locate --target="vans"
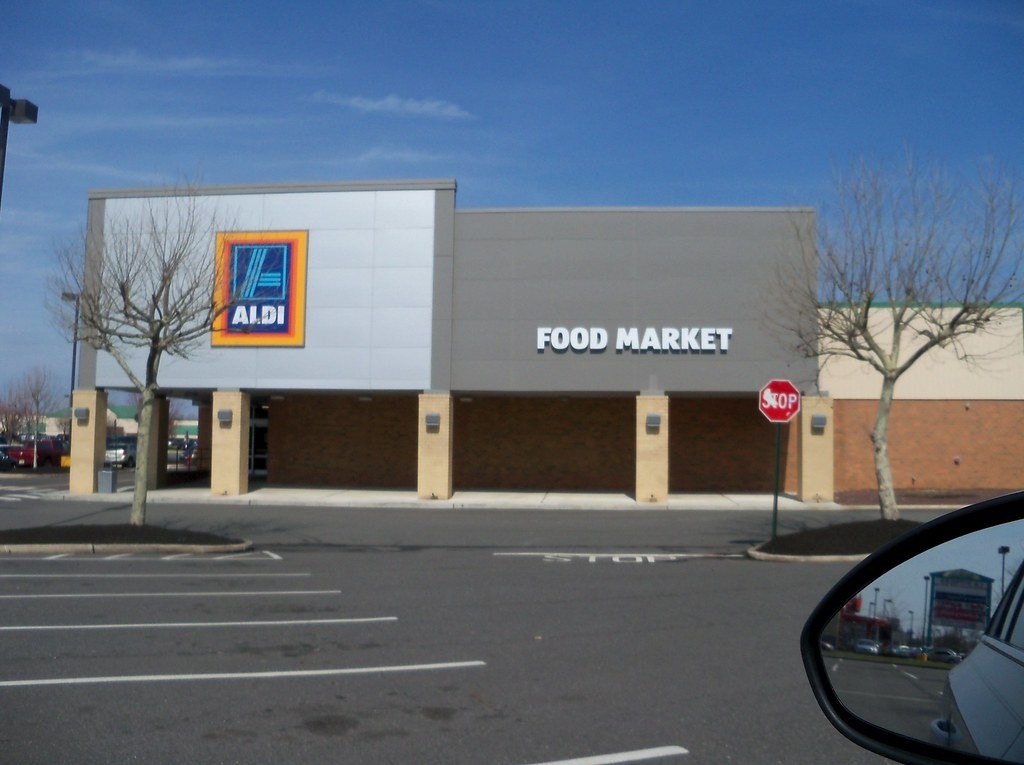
[168,438,198,462]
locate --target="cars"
[106,435,138,468]
[854,639,879,654]
[0,428,70,475]
[889,643,964,662]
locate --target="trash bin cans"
[97,469,117,493]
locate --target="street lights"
[999,546,1010,599]
[61,292,79,408]
[922,576,930,644]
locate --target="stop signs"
[758,380,800,422]
